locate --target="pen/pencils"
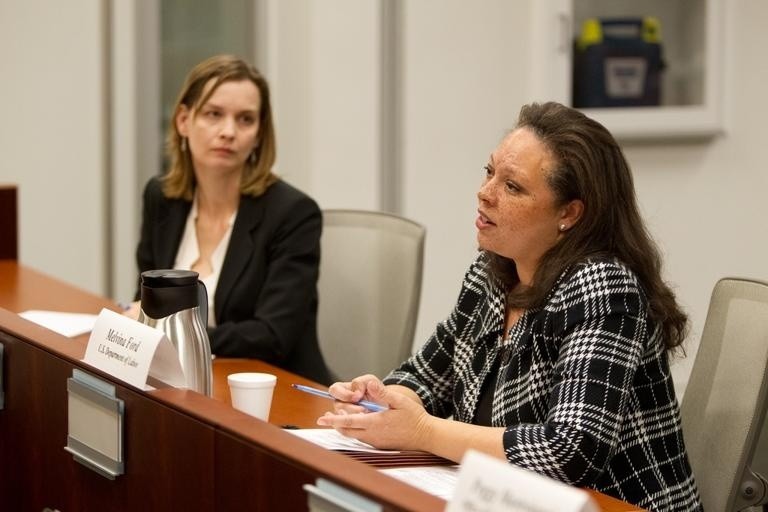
[289,383,387,412]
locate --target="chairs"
[318,209,427,387]
[680,275,768,511]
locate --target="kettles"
[136,269,215,398]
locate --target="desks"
[0,258,647,512]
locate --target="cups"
[227,373,278,423]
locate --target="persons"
[122,53,333,387]
[317,102,703,512]
